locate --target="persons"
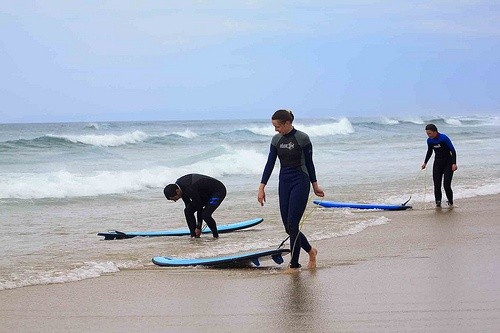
[258,110,325,274]
[164,174,227,240]
[420,123,457,210]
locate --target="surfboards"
[97,217,264,240]
[313,200,412,210]
[152,249,290,266]
